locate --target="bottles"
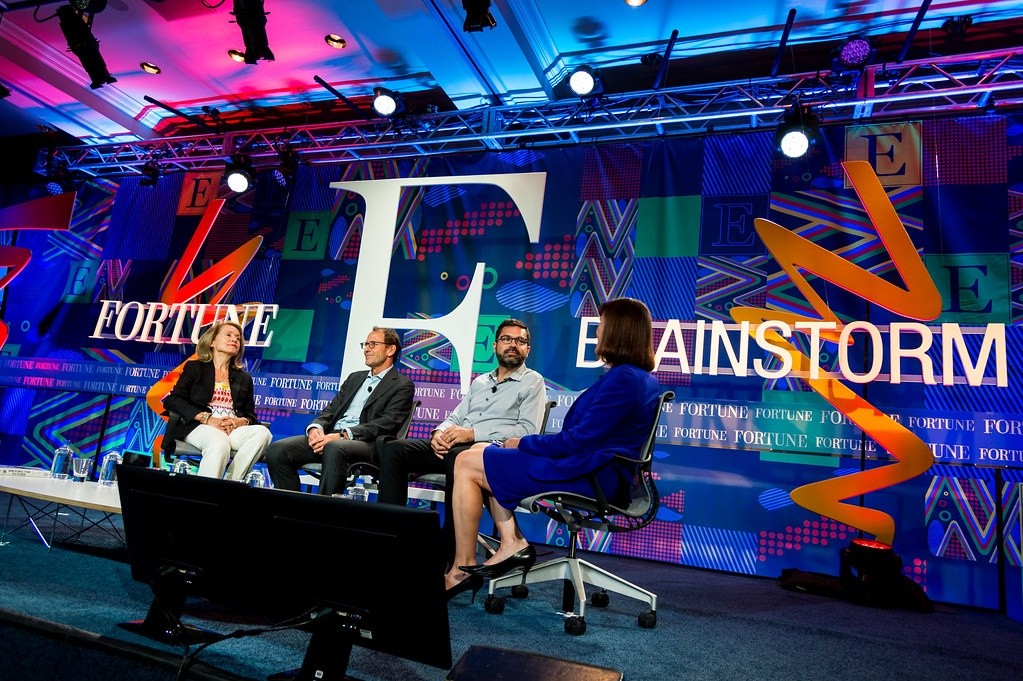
[174,456,192,475]
[348,478,369,502]
[98,447,122,490]
[50,439,74,479]
[245,464,265,488]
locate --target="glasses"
[360,341,390,350]
[496,335,528,346]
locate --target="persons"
[161,322,273,480]
[267,327,416,496]
[378,319,546,532]
[446,298,660,606]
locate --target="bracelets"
[200,411,211,424]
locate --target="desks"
[0,465,127,553]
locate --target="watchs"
[339,433,345,439]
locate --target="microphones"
[230,375,236,383]
[368,386,372,393]
[492,386,497,392]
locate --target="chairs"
[484,390,675,634]
[414,400,558,572]
[301,401,422,501]
[164,395,271,476]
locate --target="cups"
[332,493,353,500]
[72,458,91,482]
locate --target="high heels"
[447,574,484,604]
[459,544,537,585]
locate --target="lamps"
[227,163,253,192]
[272,157,298,188]
[373,86,398,116]
[774,111,809,159]
[44,174,70,195]
[569,64,599,95]
[139,160,161,188]
[839,36,871,65]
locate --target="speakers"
[447,644,625,681]
